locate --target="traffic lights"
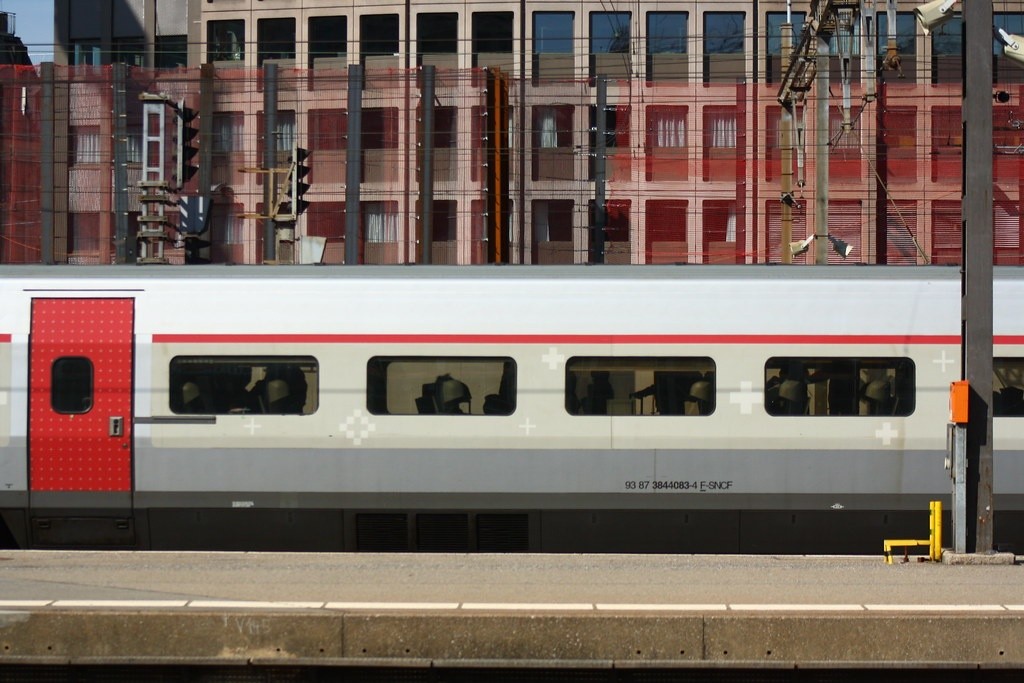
[171,98,200,190]
[287,140,311,220]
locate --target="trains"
[0,263,1024,555]
